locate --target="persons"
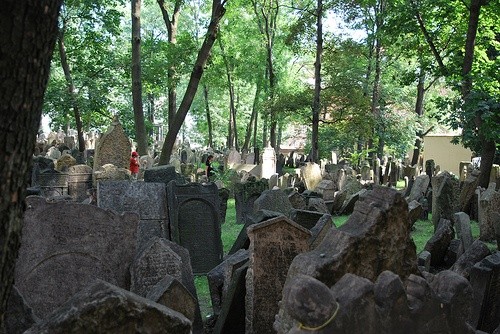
[205,155,214,184]
[130,151,141,180]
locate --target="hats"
[132,152,138,156]
